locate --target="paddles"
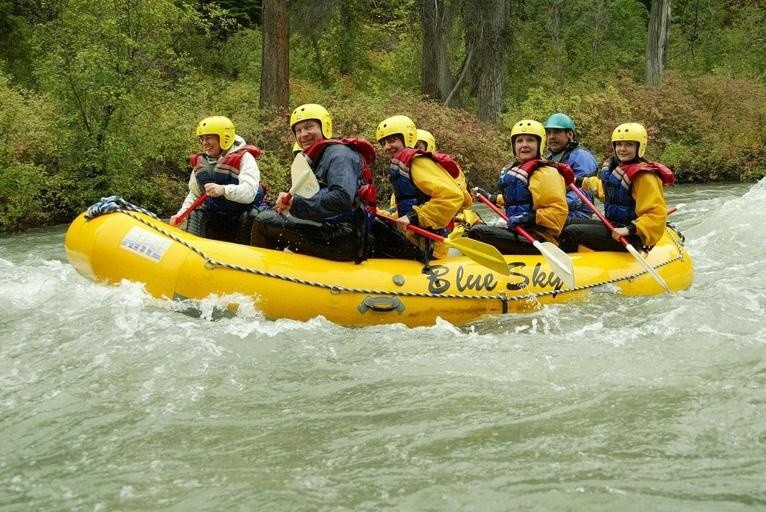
[476,194,574,289]
[376,213,509,274]
[569,182,669,294]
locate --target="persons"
[252,104,378,262]
[169,115,265,244]
[376,114,466,262]
[542,112,597,226]
[461,120,568,256]
[556,123,674,254]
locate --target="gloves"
[507,211,529,230]
[470,186,496,206]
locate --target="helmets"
[376,115,418,149]
[510,119,547,158]
[544,112,575,133]
[417,129,435,155]
[290,103,332,158]
[611,122,648,159]
[196,116,235,151]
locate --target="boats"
[63,200,697,329]
